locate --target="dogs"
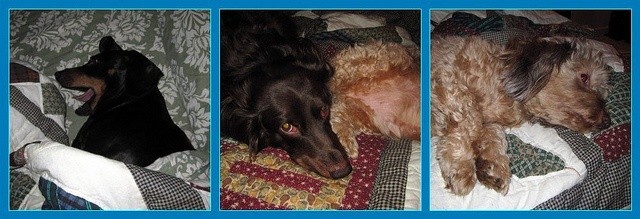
[54,35,196,167]
[220,10,353,180]
[324,31,615,200]
